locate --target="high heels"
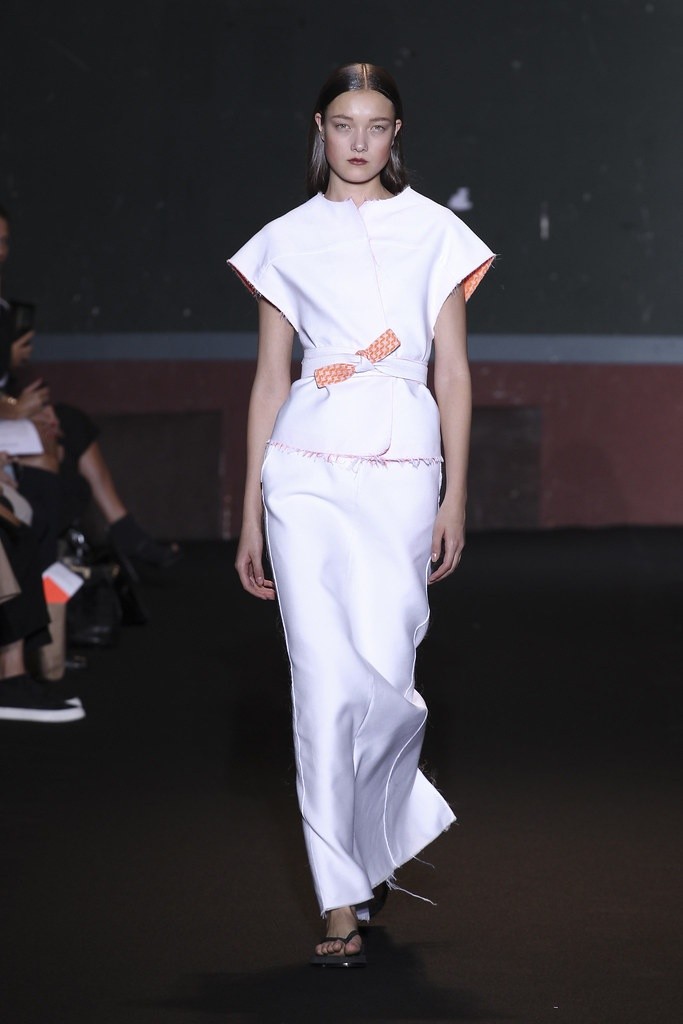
[101,513,182,584]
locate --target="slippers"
[309,929,367,968]
[366,881,389,917]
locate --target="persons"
[228,61,495,971]
[0,217,180,723]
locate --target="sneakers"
[0,672,86,723]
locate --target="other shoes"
[119,606,151,624]
